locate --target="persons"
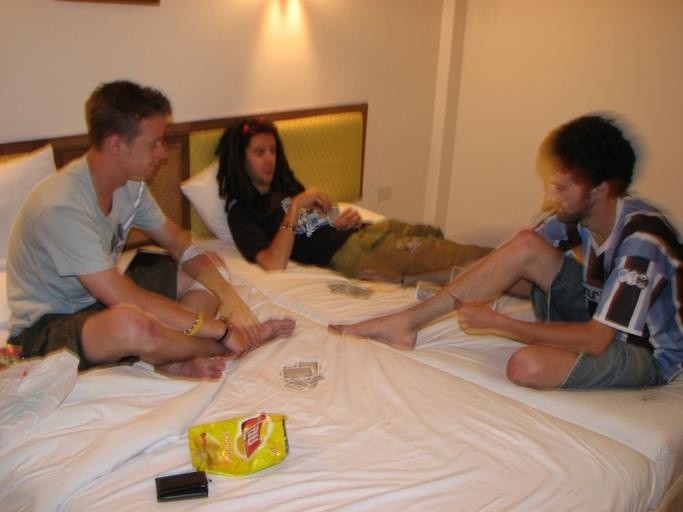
[214,117,540,298]
[5,79,296,382]
[326,112,682,391]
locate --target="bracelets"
[214,322,231,342]
[278,222,297,234]
[182,311,206,337]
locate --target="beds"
[0,101,682,511]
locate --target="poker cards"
[448,290,463,302]
[450,267,463,281]
[327,281,376,300]
[327,204,339,226]
[279,360,325,391]
[415,280,442,300]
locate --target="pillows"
[182,157,233,242]
[0,143,57,272]
